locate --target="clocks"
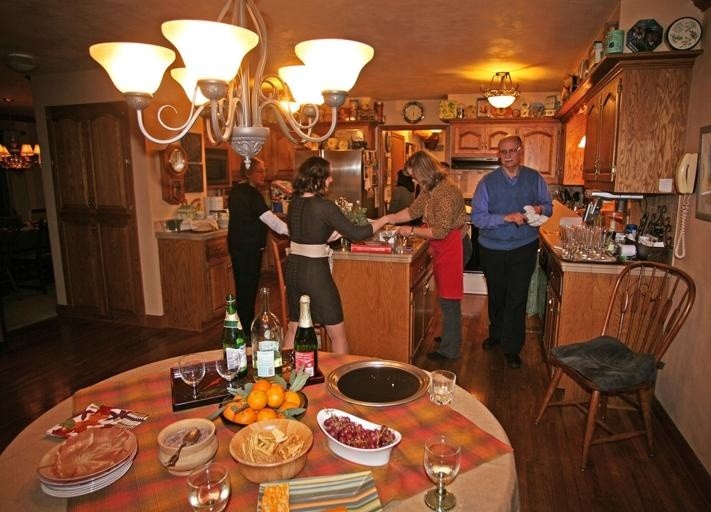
[157,142,191,205]
[401,101,426,125]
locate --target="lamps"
[85,0,377,172]
[478,71,524,111]
[0,116,42,171]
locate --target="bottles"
[163,199,227,232]
[407,232,417,251]
[570,21,625,89]
[605,198,641,261]
[294,295,318,377]
[553,188,582,211]
[251,288,284,381]
[310,99,385,151]
[220,294,249,377]
[437,96,558,117]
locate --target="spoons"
[166,427,203,469]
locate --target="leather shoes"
[504,351,522,369]
[482,335,501,351]
[427,352,449,360]
[434,336,443,344]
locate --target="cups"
[187,463,233,511]
[431,371,456,404]
[559,222,606,260]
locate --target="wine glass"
[215,349,242,393]
[381,224,394,244]
[423,435,463,510]
[180,353,208,399]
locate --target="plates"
[628,19,664,52]
[256,470,382,512]
[38,403,148,497]
[667,18,703,52]
[219,385,310,434]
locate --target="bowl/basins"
[229,418,314,482]
[156,416,218,475]
[318,405,403,467]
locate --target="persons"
[469,134,554,369]
[283,156,394,354]
[385,147,467,361]
[394,157,418,194]
[224,154,290,335]
[438,160,452,175]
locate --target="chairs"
[527,259,698,474]
[271,239,325,349]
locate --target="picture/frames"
[694,125,711,224]
[405,141,414,159]
[385,154,394,186]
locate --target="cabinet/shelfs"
[558,87,587,191]
[202,110,298,189]
[157,214,290,335]
[320,237,440,363]
[534,244,667,395]
[582,49,702,201]
[516,112,565,187]
[446,118,515,162]
[298,116,383,150]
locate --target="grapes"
[324,417,395,449]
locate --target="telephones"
[674,153,698,194]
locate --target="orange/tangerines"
[223,380,300,424]
